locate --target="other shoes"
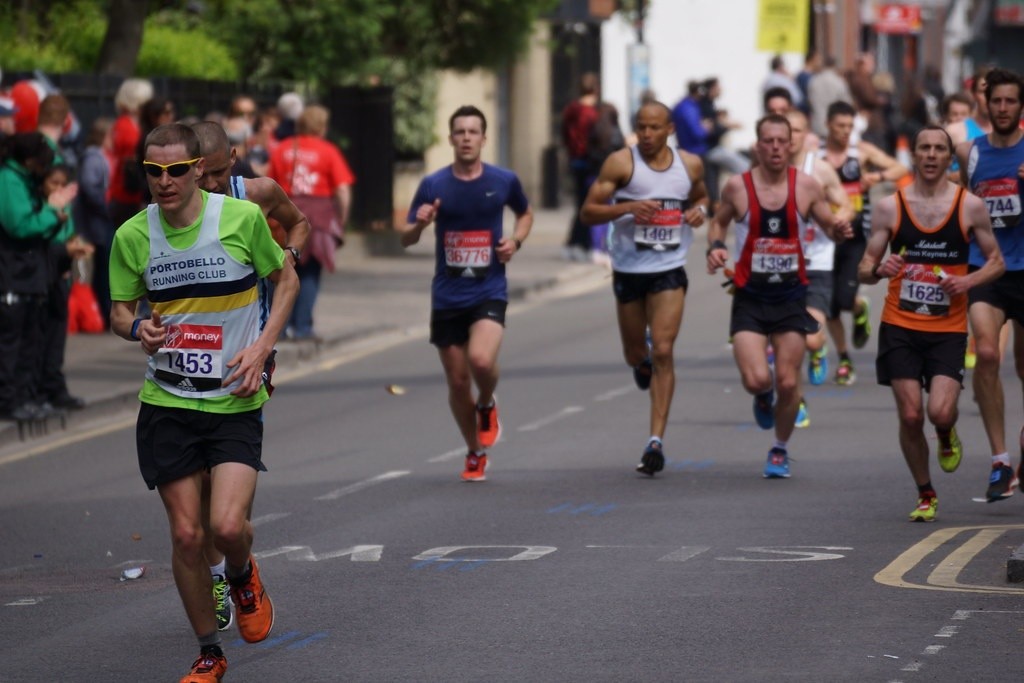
[6,396,68,425]
[49,385,89,409]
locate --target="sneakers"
[461,448,489,482]
[1016,463,1024,493]
[210,573,233,632]
[853,296,872,349]
[762,448,798,478]
[752,363,776,430]
[908,496,938,523]
[793,397,810,427]
[224,552,275,643]
[937,423,963,473]
[630,329,653,390]
[808,346,828,386]
[831,359,856,385]
[179,654,228,683]
[475,392,501,449]
[634,445,665,478]
[985,461,1020,500]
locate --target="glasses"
[142,157,201,177]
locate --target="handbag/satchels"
[66,256,107,333]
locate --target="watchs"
[696,204,708,217]
[509,238,521,250]
[872,263,883,279]
[711,241,725,248]
[284,246,302,268]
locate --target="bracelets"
[131,318,144,341]
[880,172,884,181]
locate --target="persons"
[856,126,1006,525]
[108,118,314,683]
[952,68,1024,504]
[579,99,710,476]
[0,77,357,423]
[560,71,751,264]
[706,116,855,480]
[749,50,1009,428]
[397,104,534,483]
